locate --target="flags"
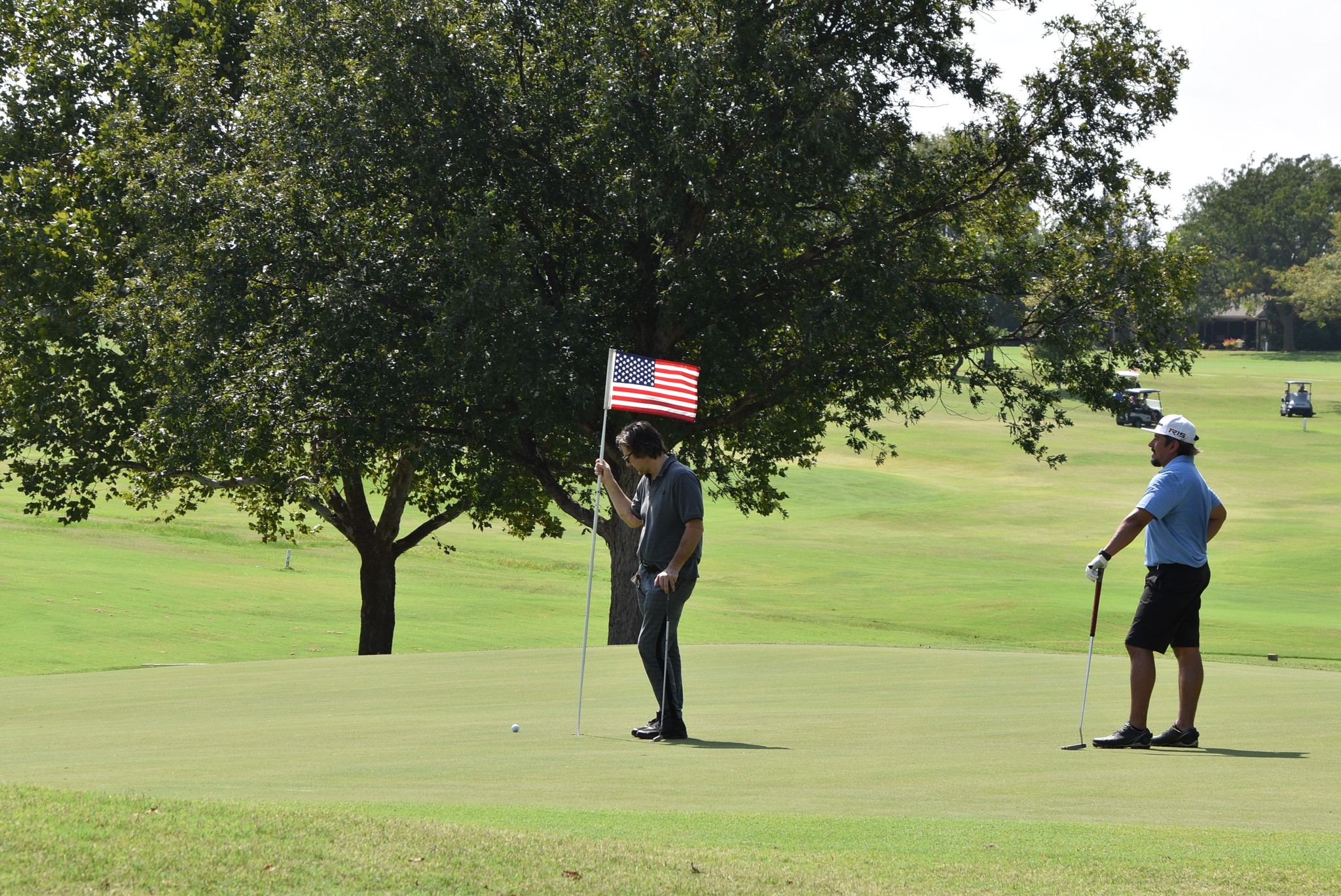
[610,350,701,423]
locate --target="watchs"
[1098,548,1112,561]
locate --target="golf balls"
[511,724,520,732]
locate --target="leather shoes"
[631,719,688,739]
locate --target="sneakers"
[1152,723,1200,747]
[1092,722,1153,750]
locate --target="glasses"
[622,452,633,463]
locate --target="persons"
[1298,385,1308,393]
[1139,392,1158,425]
[1085,413,1227,750]
[595,422,705,740]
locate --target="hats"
[1141,414,1197,444]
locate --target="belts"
[640,561,661,572]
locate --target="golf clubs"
[652,582,672,743]
[1061,567,1105,750]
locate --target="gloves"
[1085,555,1117,574]
